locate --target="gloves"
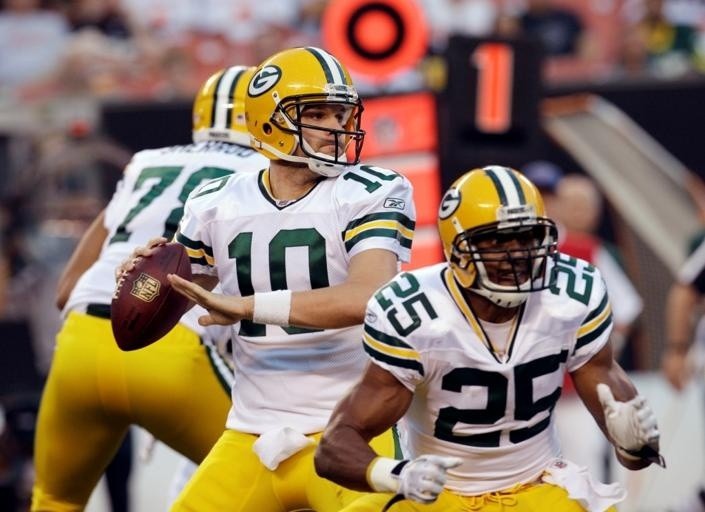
[367,454,464,503]
[596,383,661,461]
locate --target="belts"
[87,304,111,319]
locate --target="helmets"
[192,64,258,148]
[245,47,365,177]
[438,165,560,308]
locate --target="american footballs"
[111,243,192,350]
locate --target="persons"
[1,0,705,511]
